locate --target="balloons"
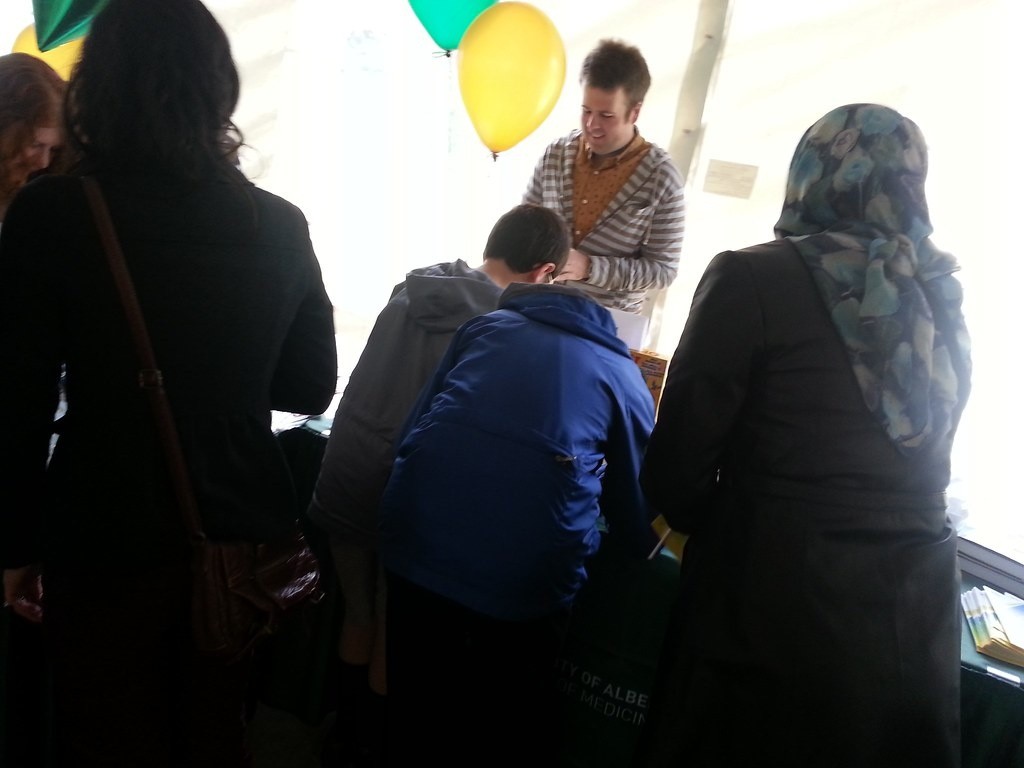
[11,19,85,82]
[408,0,500,58]
[458,1,567,162]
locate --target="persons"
[301,204,570,768]
[380,283,655,768]
[522,40,686,355]
[0,0,338,768]
[638,104,973,768]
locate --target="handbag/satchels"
[198,533,320,655]
